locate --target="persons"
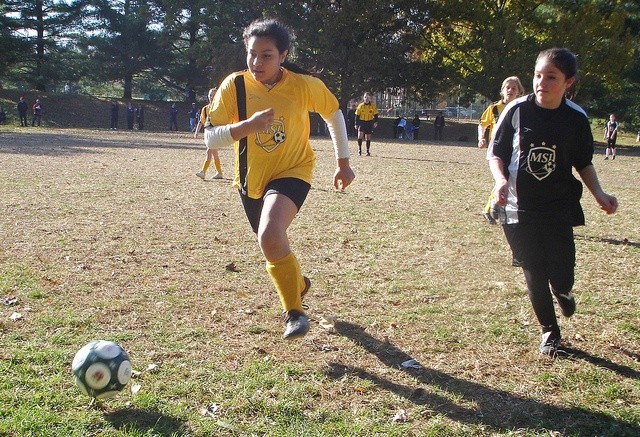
[188,103,196,132]
[204,18,356,340]
[17,96,28,127]
[65,82,70,95]
[195,109,200,122]
[0,108,6,124]
[168,102,178,131]
[193,88,224,180]
[477,75,525,225]
[434,112,445,140]
[486,47,618,356]
[109,99,118,130]
[387,108,393,116]
[136,103,144,130]
[603,114,618,160]
[354,91,379,156]
[412,114,420,140]
[398,117,407,140]
[126,101,134,130]
[392,117,400,139]
[31,99,42,127]
[347,104,357,137]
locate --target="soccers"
[70,340,131,400]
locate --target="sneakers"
[282,309,309,341]
[211,173,224,179]
[552,287,575,317]
[540,332,561,355]
[196,171,205,179]
[300,276,311,309]
